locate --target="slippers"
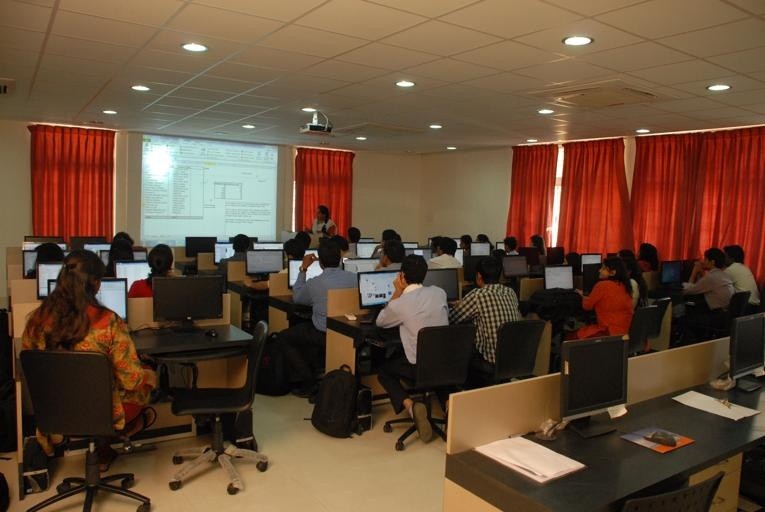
[312,364,357,438]
[253,332,292,396]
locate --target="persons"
[566,243,656,337]
[220,234,250,264]
[30,232,134,279]
[267,228,405,287]
[684,248,735,344]
[426,234,544,268]
[375,254,449,441]
[21,247,159,471]
[128,244,173,299]
[448,258,519,389]
[723,244,761,312]
[313,205,336,238]
[270,240,358,399]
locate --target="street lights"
[531,289,583,310]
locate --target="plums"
[298,122,333,138]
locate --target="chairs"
[411,402,431,442]
[97,446,117,469]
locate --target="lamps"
[473,318,545,384]
[170,319,268,495]
[701,289,750,335]
[17,348,150,512]
[385,325,480,451]
[651,297,672,326]
[618,469,725,512]
[623,303,658,356]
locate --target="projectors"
[298,122,333,138]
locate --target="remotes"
[643,428,677,449]
[206,328,219,340]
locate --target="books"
[472,436,587,484]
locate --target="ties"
[22,241,221,331]
[562,333,630,439]
[730,311,765,383]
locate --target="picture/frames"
[230,280,265,306]
[445,381,763,511]
[267,295,312,334]
[126,323,255,359]
[326,315,389,408]
[174,258,194,277]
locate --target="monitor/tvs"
[661,259,683,285]
[403,238,463,267]
[245,249,284,282]
[343,259,381,275]
[547,246,565,265]
[358,270,402,325]
[288,259,323,289]
[681,259,695,283]
[496,242,506,251]
[185,231,382,258]
[730,311,765,383]
[501,255,528,278]
[472,436,587,484]
[562,333,630,439]
[579,254,602,273]
[518,246,541,271]
[22,241,221,331]
[544,266,574,290]
[470,242,491,256]
[582,264,603,297]
